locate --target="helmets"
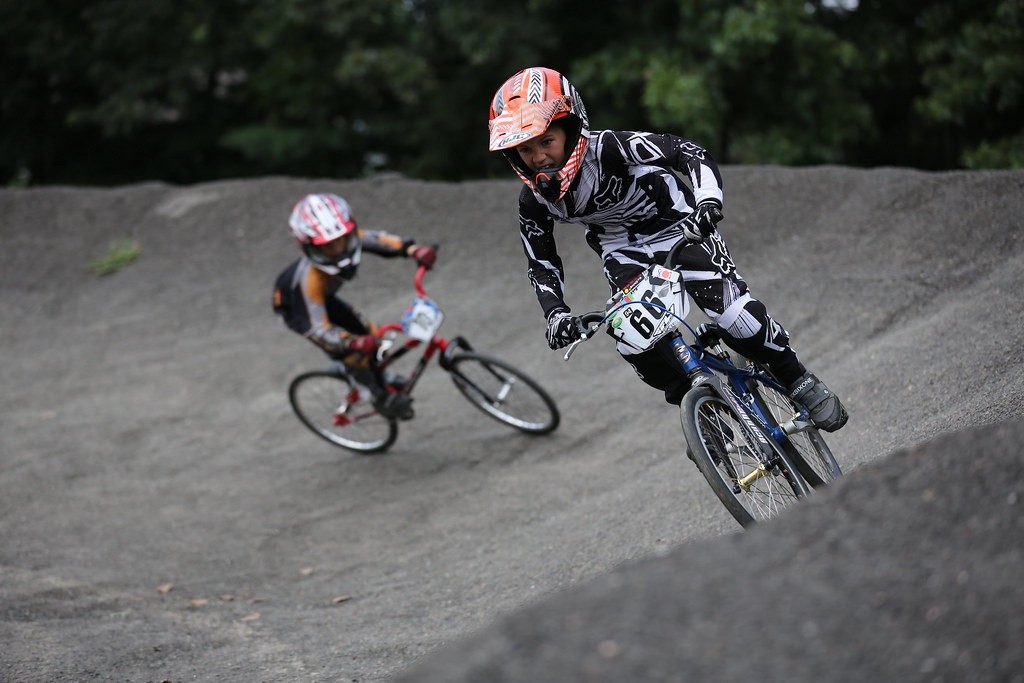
[289,193,361,280]
[489,67,590,205]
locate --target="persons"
[268,191,437,421]
[486,64,849,471]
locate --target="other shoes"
[384,375,407,391]
[372,391,415,421]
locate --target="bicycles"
[287,241,562,456]
[555,205,845,532]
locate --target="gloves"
[680,203,719,246]
[545,307,581,352]
[350,335,379,356]
[408,245,437,271]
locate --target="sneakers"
[686,412,734,460]
[788,370,841,433]
